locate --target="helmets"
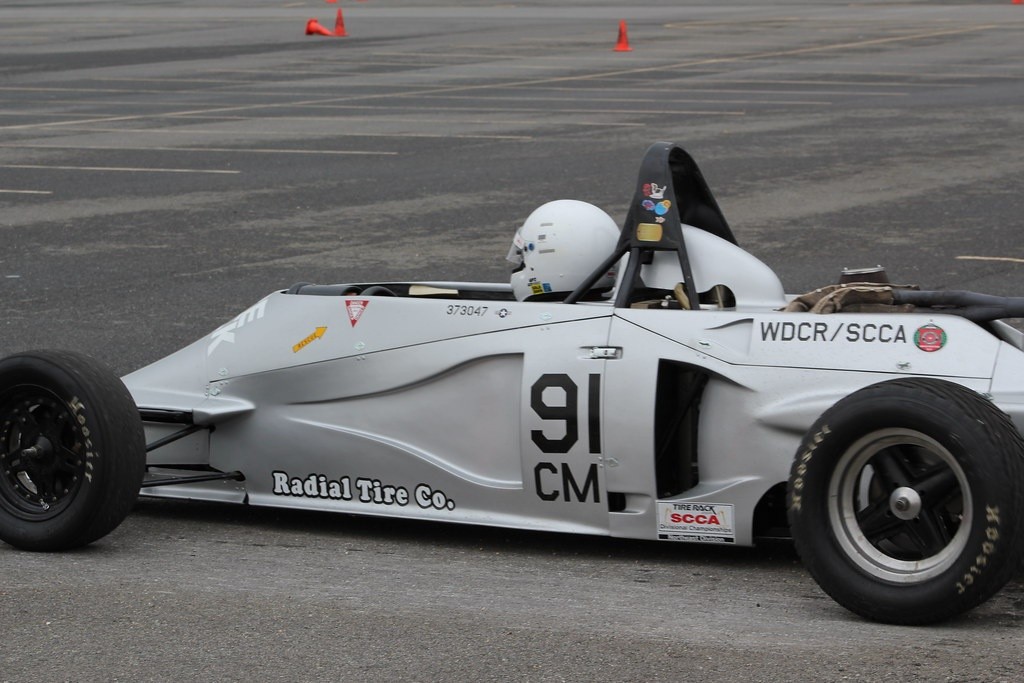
[511,199,621,302]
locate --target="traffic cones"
[613,18,633,53]
[304,19,332,37]
[330,6,350,38]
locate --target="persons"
[505,200,622,302]
